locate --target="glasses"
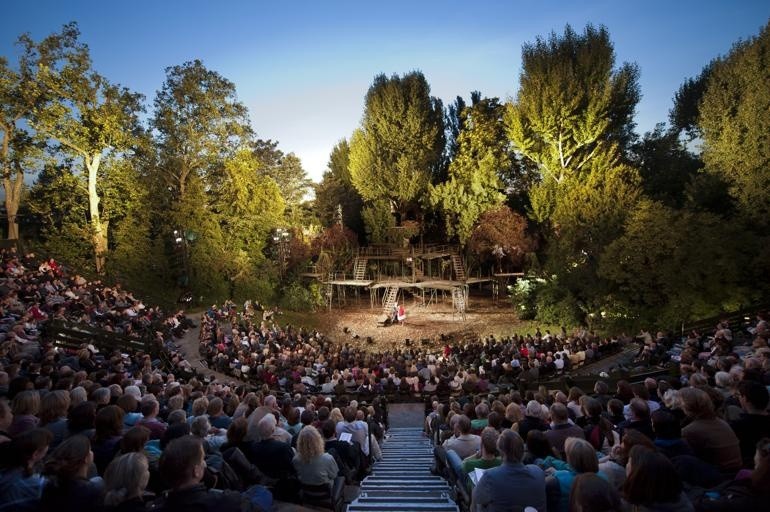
[735,393,742,398]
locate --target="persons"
[421,383,770,511]
[390,300,399,324]
[631,308,770,384]
[307,325,631,384]
[0,237,199,511]
[199,373,390,512]
[396,302,407,327]
[198,300,307,373]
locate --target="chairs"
[298,476,345,512]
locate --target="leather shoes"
[429,465,449,480]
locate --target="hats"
[565,437,598,463]
[240,487,273,512]
